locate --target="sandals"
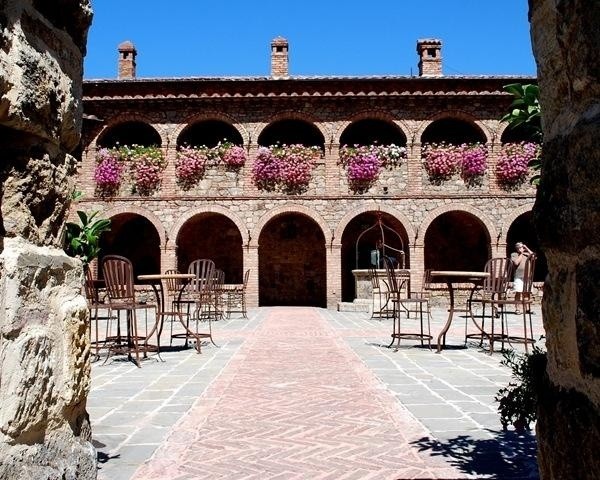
[515,310,521,315]
[526,309,535,314]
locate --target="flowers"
[423,142,540,189]
[92,142,166,195]
[335,139,409,194]
[249,141,328,193]
[174,141,245,189]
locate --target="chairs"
[79,259,115,359]
[100,256,166,368]
[461,255,513,356]
[371,248,434,351]
[162,268,255,320]
[498,254,539,353]
[175,258,219,354]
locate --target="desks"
[426,267,490,354]
[141,273,198,355]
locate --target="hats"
[515,241,523,248]
